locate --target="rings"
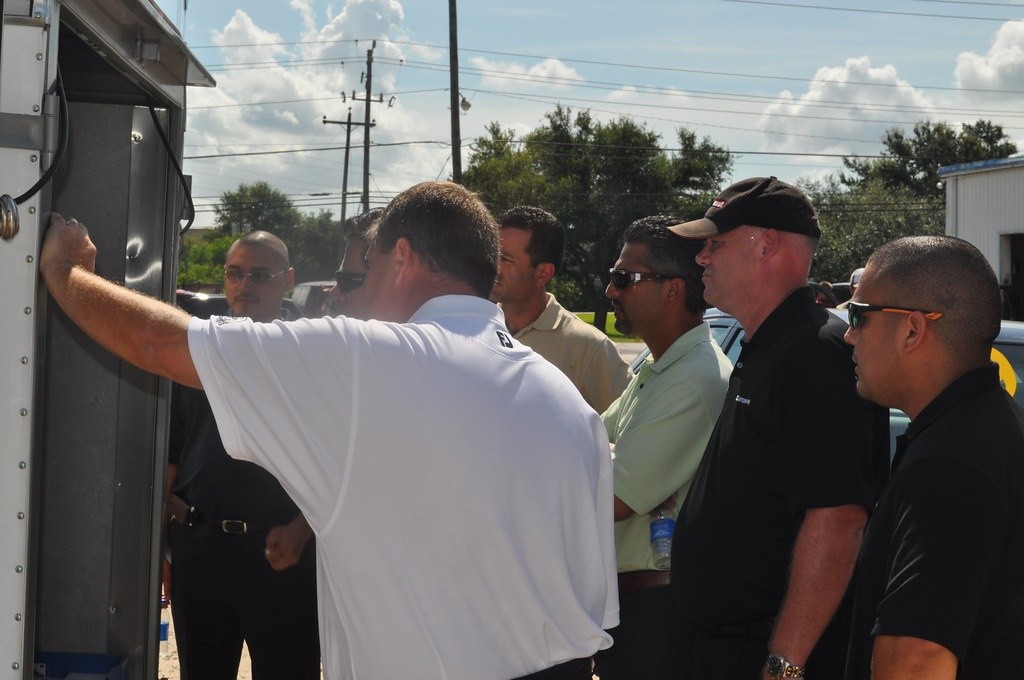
[66,218,79,225]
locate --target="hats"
[666,175,822,240]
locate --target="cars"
[616,301,1023,478]
[174,278,346,327]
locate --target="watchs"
[764,652,807,680]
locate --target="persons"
[161,229,322,680]
[329,205,383,321]
[843,235,1023,680]
[592,215,735,680]
[667,175,891,680]
[39,178,622,679]
[816,281,844,309]
[488,205,636,416]
[835,268,865,309]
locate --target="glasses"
[608,267,681,289]
[845,301,945,330]
[333,270,366,294]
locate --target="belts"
[188,513,272,536]
